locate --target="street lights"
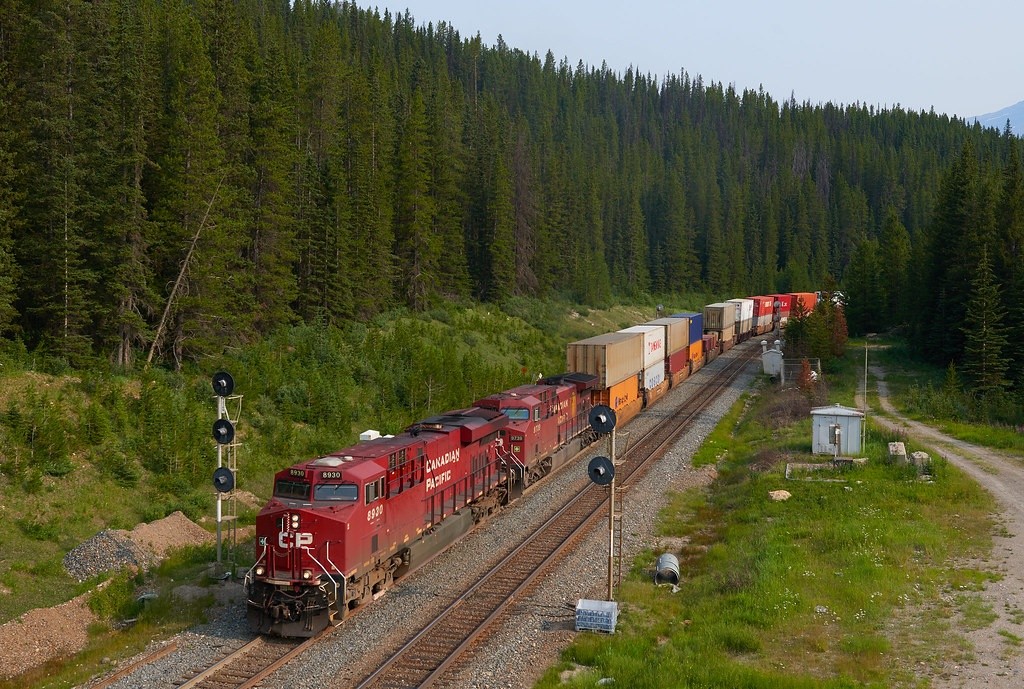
[863,333,876,453]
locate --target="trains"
[240,275,848,643]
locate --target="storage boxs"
[566,292,817,413]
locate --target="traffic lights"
[212,371,234,397]
[587,457,615,486]
[212,420,234,444]
[589,405,616,432]
[212,467,234,493]
